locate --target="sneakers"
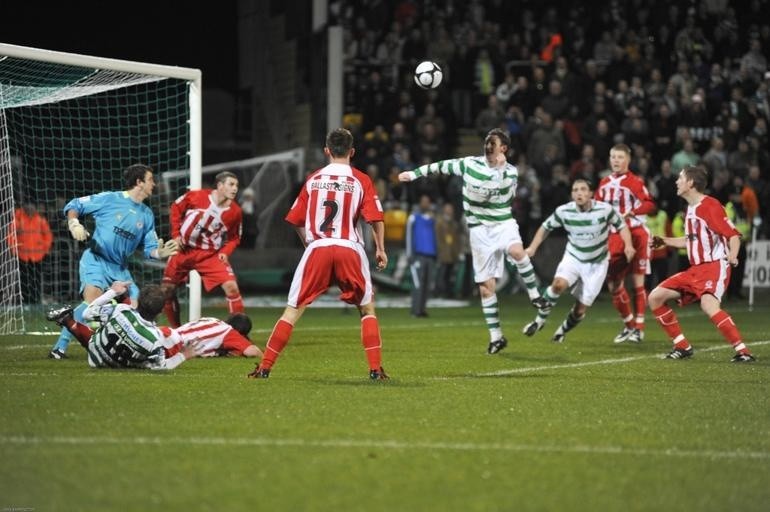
[551,327,565,343]
[522,321,544,338]
[486,335,507,354]
[614,327,635,343]
[628,329,645,343]
[369,366,390,381]
[664,344,694,360]
[730,351,755,363]
[247,362,271,379]
[530,295,552,309]
[48,348,69,360]
[47,305,74,327]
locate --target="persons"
[249,128,388,378]
[725,184,753,301]
[9,198,54,305]
[162,171,248,334]
[497,9,595,202]
[340,6,496,126]
[399,128,551,355]
[434,204,465,296]
[460,211,472,296]
[645,197,672,294]
[352,126,444,198]
[597,143,653,334]
[705,6,768,222]
[672,200,691,273]
[406,196,436,316]
[83,280,205,373]
[46,305,265,368]
[50,164,180,360]
[634,165,680,212]
[523,175,636,343]
[596,5,706,165]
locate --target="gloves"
[68,218,90,241]
[157,239,178,259]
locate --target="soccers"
[414,60,443,90]
